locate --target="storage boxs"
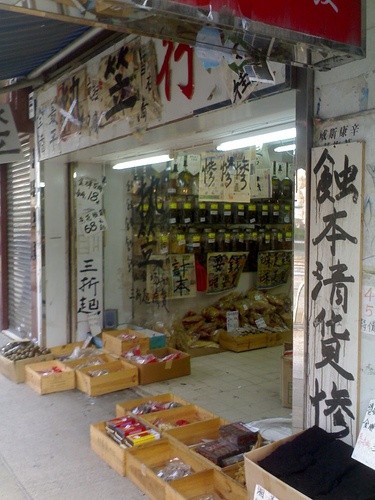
[0,328,312,500]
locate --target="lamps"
[110,150,178,170]
